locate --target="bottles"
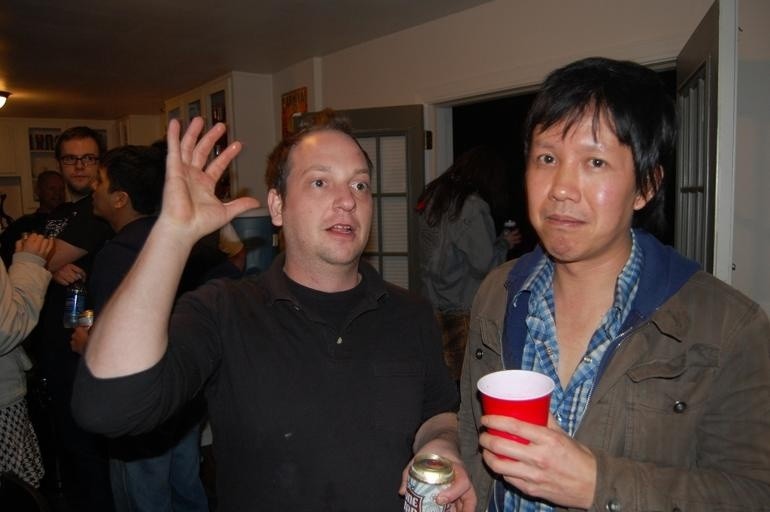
[504,220,515,234]
[62,274,85,328]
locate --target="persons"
[68,117,479,512]
[457,56,769,510]
[0,126,247,511]
[420,140,522,376]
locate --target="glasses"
[61,153,99,166]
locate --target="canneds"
[76,310,94,334]
[404,453,456,512]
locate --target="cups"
[476,370,555,461]
[29,134,60,150]
